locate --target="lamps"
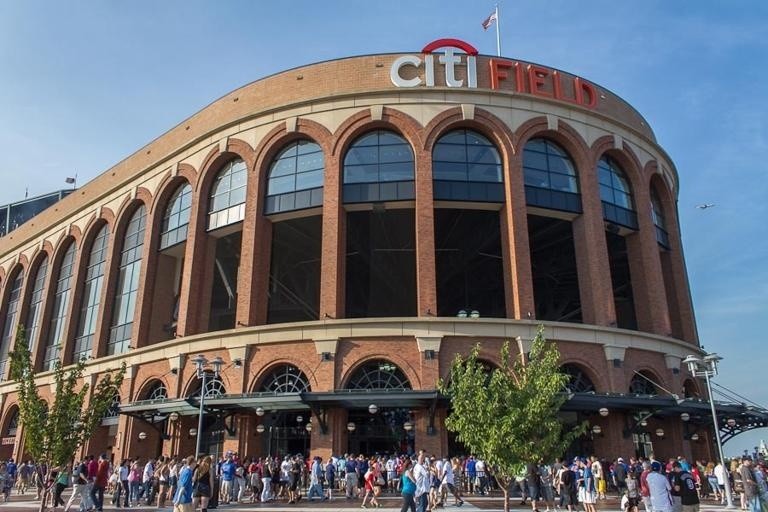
[457,310,467,318]
[170,411,179,421]
[137,432,146,440]
[254,402,413,434]
[189,427,197,436]
[470,310,480,317]
[591,407,738,441]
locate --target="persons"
[1,447,504,512]
[513,454,767,512]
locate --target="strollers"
[625,488,639,512]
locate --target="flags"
[482,10,498,32]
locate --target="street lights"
[681,351,737,509]
[191,354,226,463]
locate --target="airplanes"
[693,203,714,210]
